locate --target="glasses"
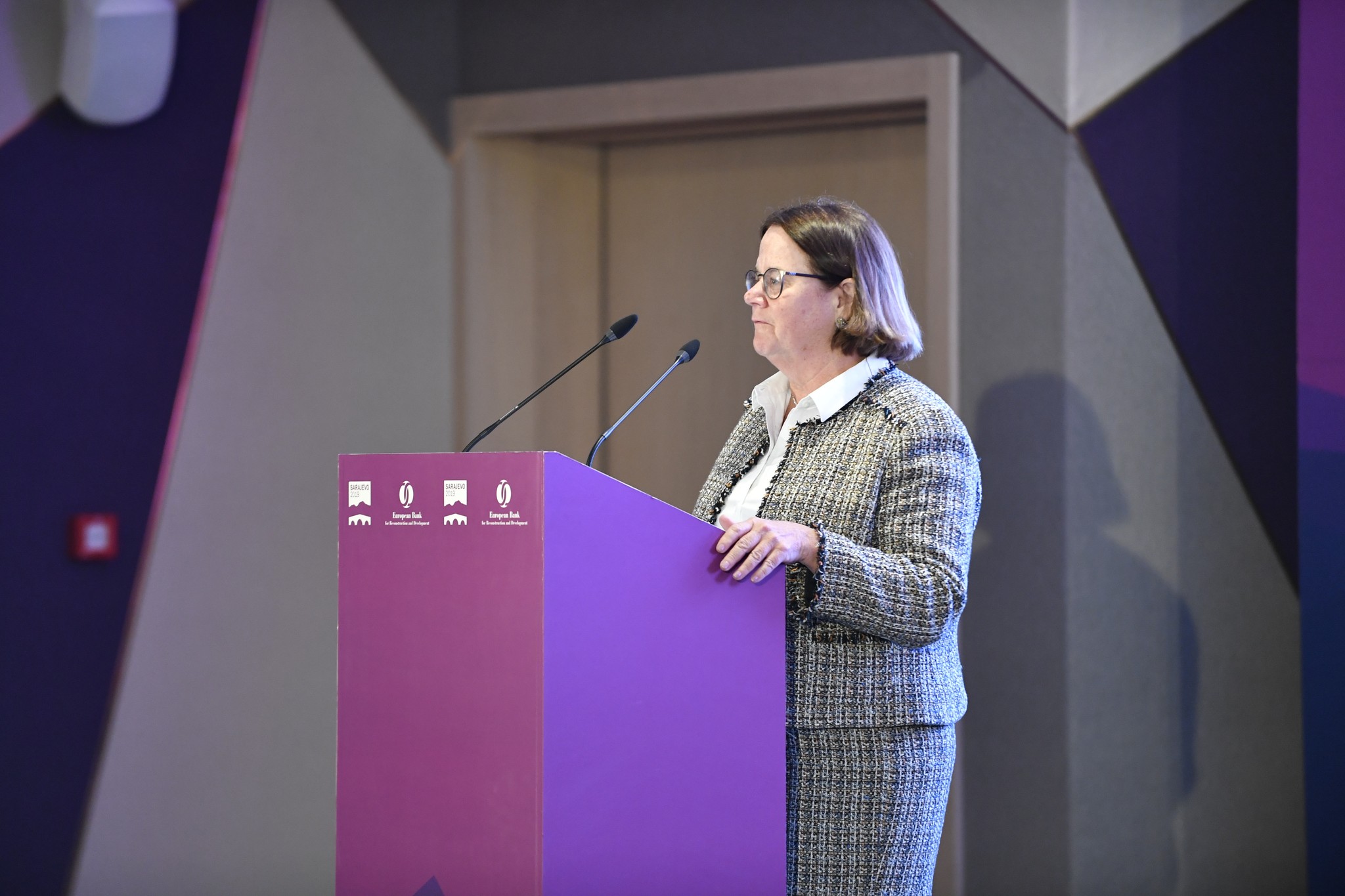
[745,268,824,299]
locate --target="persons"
[688,198,982,896]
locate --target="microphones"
[586,340,701,468]
[462,314,638,452]
